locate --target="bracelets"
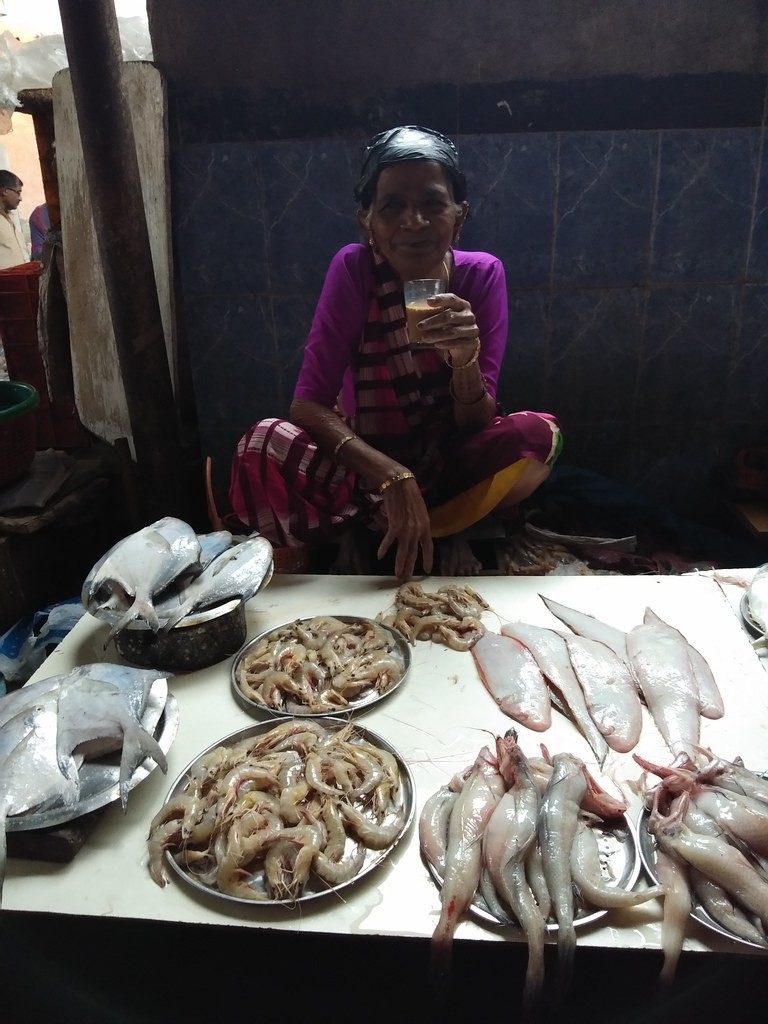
[449,373,488,409]
[443,334,482,369]
[333,435,362,472]
[378,473,416,495]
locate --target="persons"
[0,169,30,270]
[229,124,563,580]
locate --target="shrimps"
[234,614,406,715]
[148,719,408,935]
[397,582,491,652]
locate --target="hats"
[352,124,468,203]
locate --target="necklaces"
[441,260,450,294]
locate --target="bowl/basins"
[80,564,247,673]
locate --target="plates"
[160,715,416,905]
[637,769,767,954]
[1,687,181,834]
[231,613,413,717]
[419,755,641,931]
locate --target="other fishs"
[473,592,727,770]
[747,561,768,653]
[81,515,274,652]
[421,731,768,1009]
[2,662,175,897]
